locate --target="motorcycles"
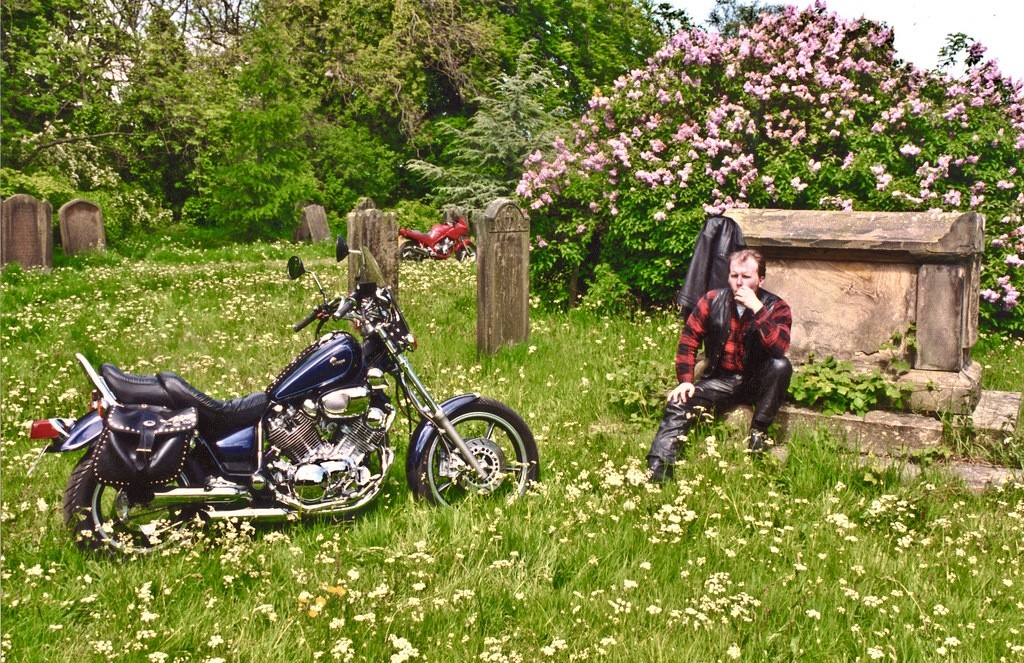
[397,217,478,262]
[25,233,539,564]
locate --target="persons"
[646,249,793,484]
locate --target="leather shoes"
[749,437,766,460]
[638,455,676,484]
